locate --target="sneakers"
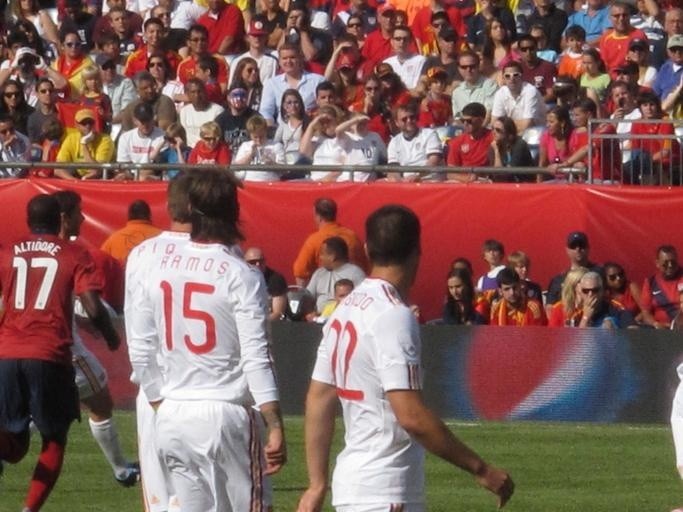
[116,458,142,483]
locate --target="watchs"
[583,315,591,322]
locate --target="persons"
[319,278,355,323]
[293,201,515,512]
[563,0,614,50]
[630,0,667,59]
[545,229,610,305]
[114,103,167,181]
[176,23,230,99]
[546,96,625,184]
[188,121,232,166]
[292,196,371,289]
[275,89,307,167]
[423,10,470,58]
[484,18,511,71]
[324,32,378,82]
[303,80,353,136]
[515,36,558,97]
[0,194,119,512]
[230,115,288,183]
[94,29,131,73]
[0,112,31,178]
[624,36,657,91]
[478,240,506,291]
[447,102,496,184]
[653,7,683,72]
[528,23,559,66]
[552,74,604,120]
[607,81,644,185]
[149,122,193,180]
[54,108,116,180]
[11,0,57,45]
[331,51,367,115]
[566,271,640,329]
[304,235,368,318]
[374,25,428,93]
[212,80,267,158]
[50,29,95,107]
[242,246,289,323]
[13,17,58,66]
[537,105,580,185]
[180,77,226,150]
[488,60,548,140]
[557,23,586,79]
[33,79,80,129]
[56,0,101,56]
[527,0,569,53]
[124,17,183,82]
[604,56,654,113]
[229,56,264,115]
[124,168,192,512]
[231,18,281,86]
[598,2,648,82]
[487,116,538,184]
[35,115,77,179]
[100,198,166,272]
[47,188,142,487]
[251,0,289,37]
[2,47,69,108]
[652,33,683,104]
[627,91,683,188]
[394,9,411,27]
[257,42,326,129]
[467,0,518,52]
[420,32,464,88]
[418,66,452,142]
[576,48,612,103]
[601,261,640,330]
[506,249,544,304]
[348,76,392,152]
[548,266,590,328]
[147,51,187,115]
[453,49,501,126]
[346,12,369,54]
[150,4,190,55]
[364,2,422,60]
[108,6,148,63]
[0,30,32,81]
[128,164,289,512]
[276,4,335,64]
[639,242,683,329]
[172,54,226,107]
[488,266,549,328]
[449,257,489,321]
[196,0,250,58]
[298,104,348,181]
[76,65,113,134]
[93,51,137,147]
[388,106,444,184]
[122,71,176,134]
[443,270,488,326]
[0,79,36,140]
[334,111,388,183]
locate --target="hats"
[566,230,587,246]
[311,10,335,29]
[462,30,486,45]
[334,49,360,70]
[628,38,646,50]
[229,81,248,92]
[249,15,269,36]
[667,34,683,49]
[94,52,115,67]
[375,3,398,16]
[427,66,448,78]
[439,26,457,40]
[610,60,640,73]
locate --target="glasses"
[133,104,155,122]
[607,271,624,280]
[286,98,301,106]
[101,61,115,69]
[459,118,472,124]
[629,47,642,53]
[366,87,378,92]
[520,46,534,52]
[75,108,95,123]
[490,125,503,132]
[140,83,158,94]
[391,36,410,42]
[582,286,599,295]
[149,61,165,70]
[158,11,172,19]
[401,114,416,122]
[163,135,174,144]
[203,136,216,143]
[347,23,362,28]
[611,13,628,18]
[188,35,206,43]
[3,91,19,98]
[431,23,444,28]
[40,87,53,94]
[1,126,14,137]
[81,120,94,127]
[457,63,478,69]
[504,72,519,80]
[248,258,265,266]
[65,41,81,49]
[247,66,260,74]
